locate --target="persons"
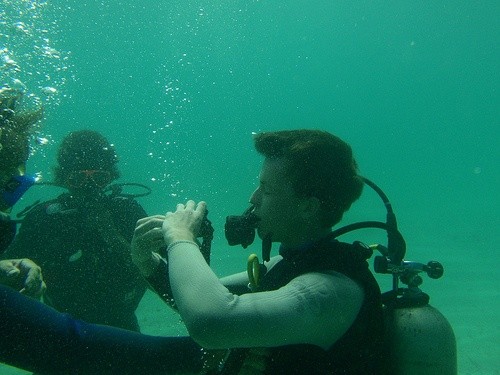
[0,129,381,375]
[0,88,48,297]
[0,129,167,334]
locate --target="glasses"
[65,169,110,188]
